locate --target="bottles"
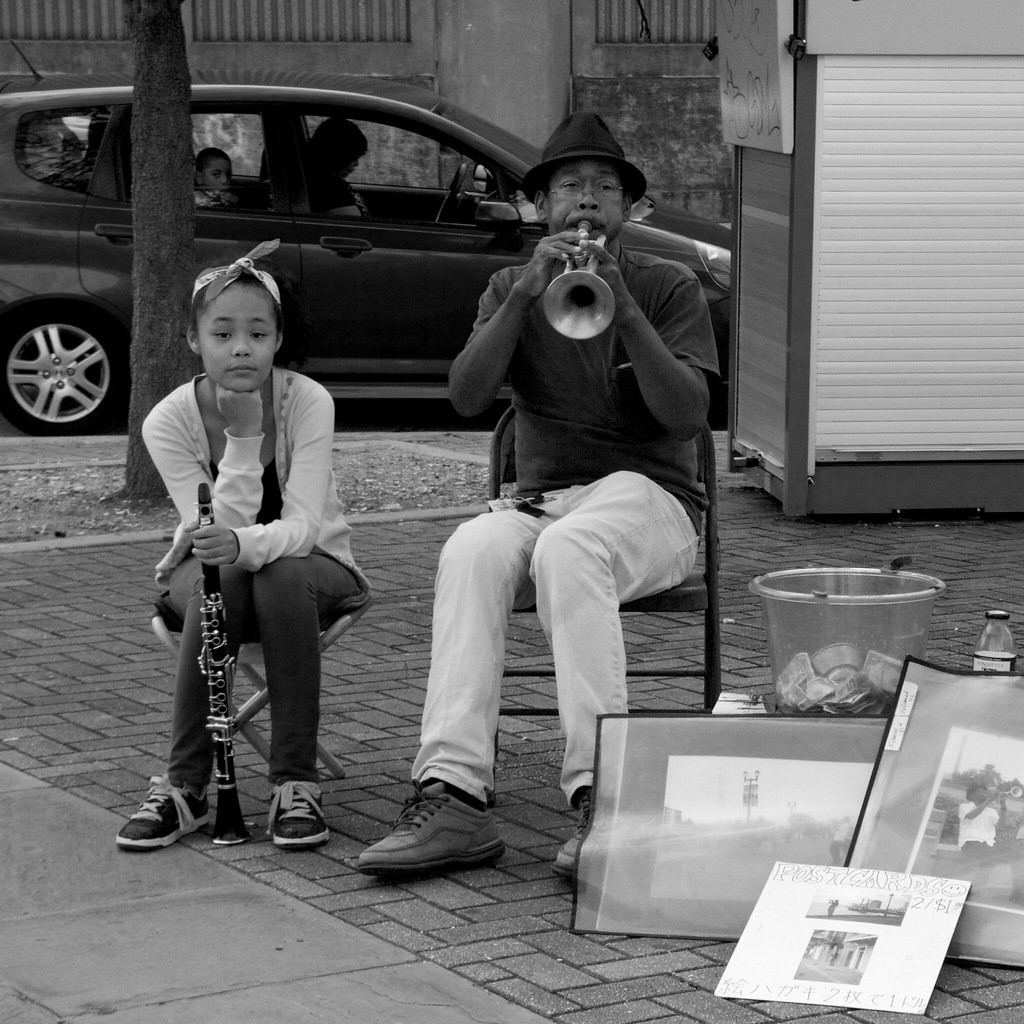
[970,610,1016,673]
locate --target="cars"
[1,36,739,437]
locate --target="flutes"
[196,482,254,846]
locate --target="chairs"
[486,396,727,757]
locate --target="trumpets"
[541,220,616,341]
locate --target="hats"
[524,111,647,203]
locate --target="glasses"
[548,180,626,199]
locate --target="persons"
[117,257,372,853]
[306,117,376,220]
[827,899,839,918]
[957,783,1024,907]
[193,147,239,213]
[354,113,720,881]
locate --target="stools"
[149,593,378,787]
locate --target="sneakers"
[115,787,208,851]
[270,780,329,848]
[553,791,591,876]
[358,783,504,877]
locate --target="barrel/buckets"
[745,566,947,717]
